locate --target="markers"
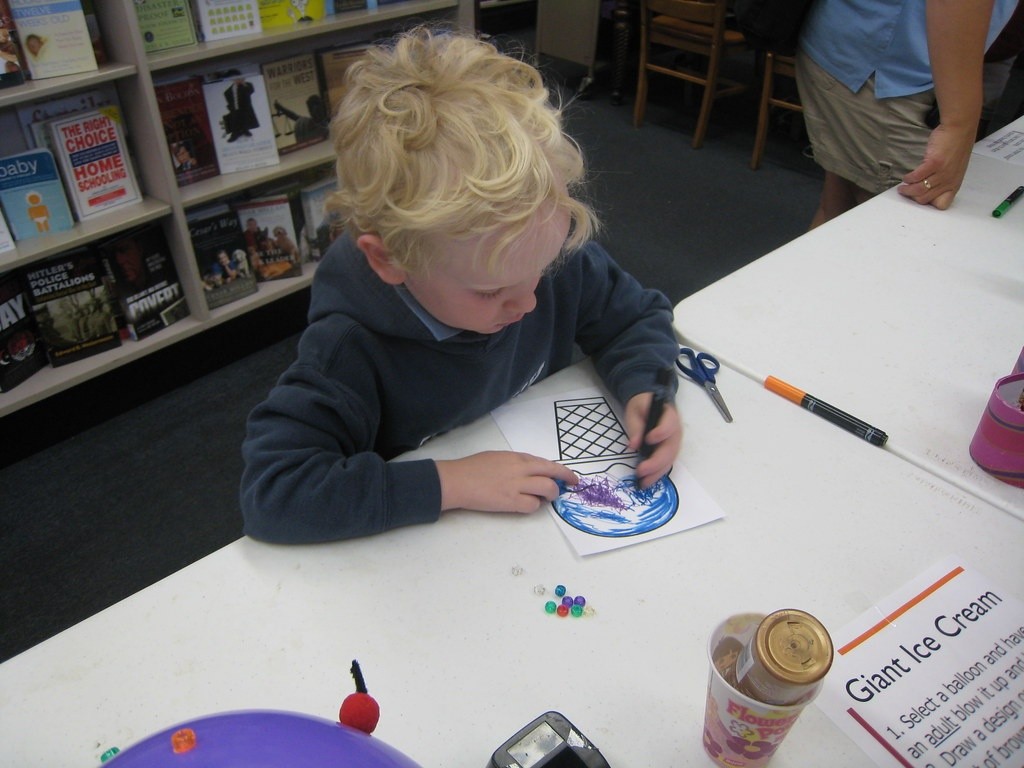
[634,366,671,493]
[992,186,1024,218]
[765,375,888,447]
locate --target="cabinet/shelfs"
[532,0,632,101]
[0,0,476,420]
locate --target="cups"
[701,613,825,768]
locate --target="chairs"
[751,54,803,170]
[632,0,744,149]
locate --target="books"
[0,0,396,390]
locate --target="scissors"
[675,348,733,423]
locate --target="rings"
[922,179,932,189]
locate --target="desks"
[0,114,1023,768]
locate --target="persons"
[214,250,235,283]
[243,218,264,268]
[202,263,224,291]
[172,142,198,174]
[238,22,685,545]
[795,0,1018,230]
